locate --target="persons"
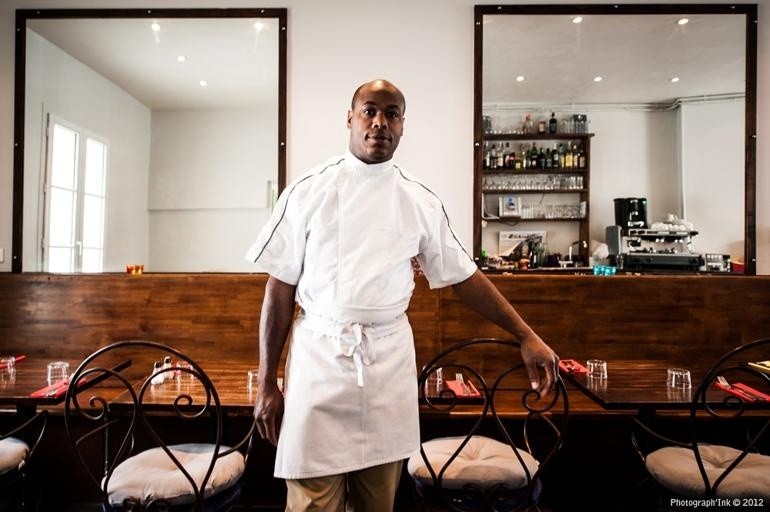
[246,80,559,512]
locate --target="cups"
[483,173,582,190]
[175,362,195,381]
[1,374,16,388]
[585,360,608,379]
[247,369,262,393]
[667,369,691,390]
[47,362,68,383]
[0,355,17,375]
[422,365,444,385]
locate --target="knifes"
[463,370,477,395]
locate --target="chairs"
[63,340,258,512]
[0,406,52,490]
[0,272,770,422]
[409,336,570,511]
[627,335,770,512]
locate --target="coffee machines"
[607,197,704,270]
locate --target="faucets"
[556,239,589,267]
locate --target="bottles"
[490,144,497,169]
[514,158,521,170]
[549,111,558,134]
[545,148,552,169]
[572,145,578,168]
[483,141,490,169]
[565,141,573,168]
[162,356,174,379]
[531,142,538,168]
[496,142,504,168]
[521,152,526,168]
[538,147,546,169]
[537,120,546,134]
[579,144,586,168]
[503,142,511,170]
[559,144,564,170]
[150,361,164,385]
[552,143,559,169]
[526,149,531,168]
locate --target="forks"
[717,375,757,401]
[46,372,73,397]
[456,372,470,395]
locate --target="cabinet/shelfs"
[476,132,597,268]
